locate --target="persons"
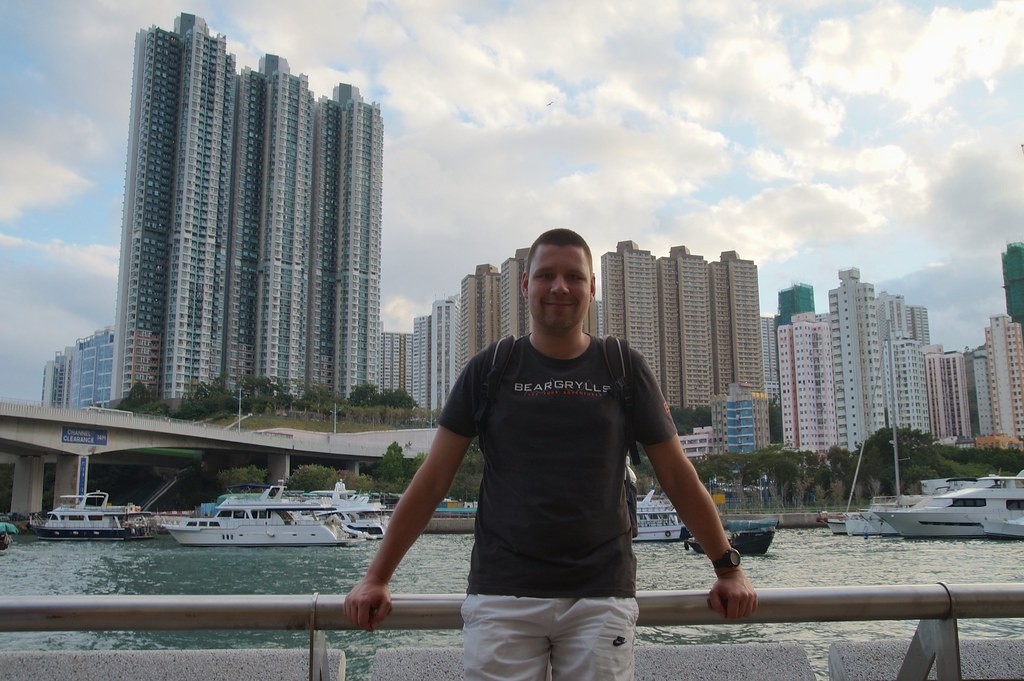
[344,229,758,681]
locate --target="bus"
[82,407,134,418]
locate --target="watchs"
[712,548,741,569]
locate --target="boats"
[632,486,687,542]
[31,489,161,541]
[680,522,776,556]
[160,480,391,547]
[816,320,1024,542]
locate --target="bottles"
[623,455,636,487]
